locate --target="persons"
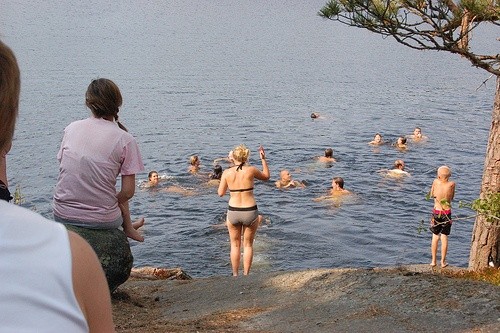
[146,113,429,225]
[0,40,118,333]
[430,166,455,268]
[217,145,270,276]
[53,78,145,242]
